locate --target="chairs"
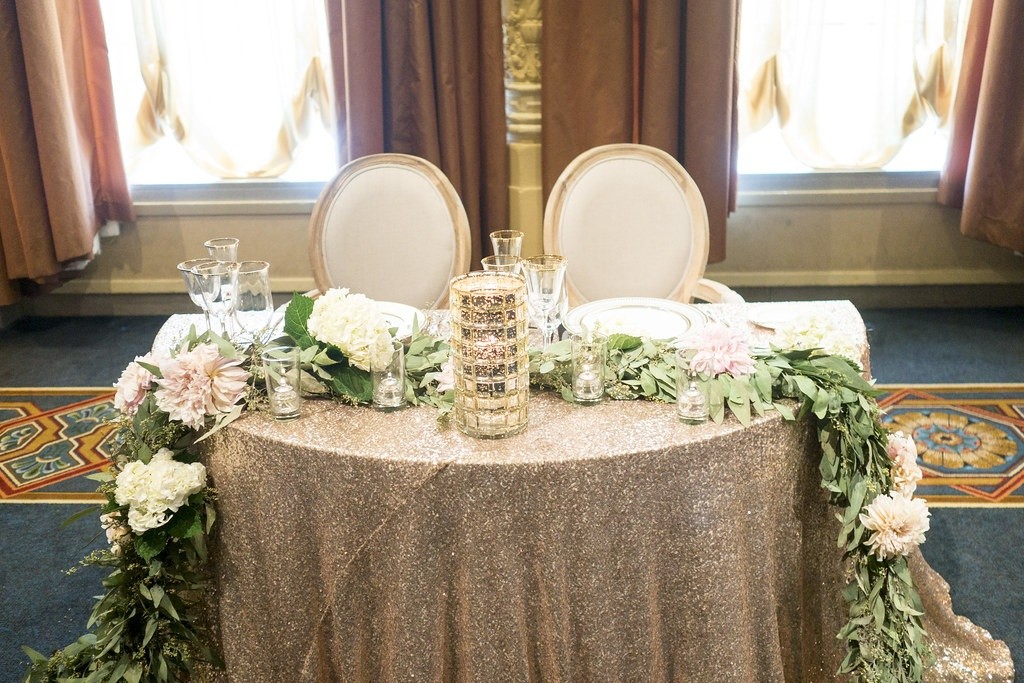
[271,154,472,309]
[543,143,744,304]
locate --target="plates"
[561,296,708,349]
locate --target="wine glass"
[178,257,221,343]
[190,261,241,336]
[525,264,568,344]
[227,261,274,343]
[521,256,567,353]
[203,237,240,338]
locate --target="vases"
[449,272,529,440]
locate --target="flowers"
[16,286,932,683]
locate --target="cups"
[674,349,710,425]
[569,333,608,406]
[260,345,302,424]
[481,254,521,277]
[370,341,408,413]
[450,270,528,439]
[489,229,524,276]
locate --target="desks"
[152,298,1018,683]
